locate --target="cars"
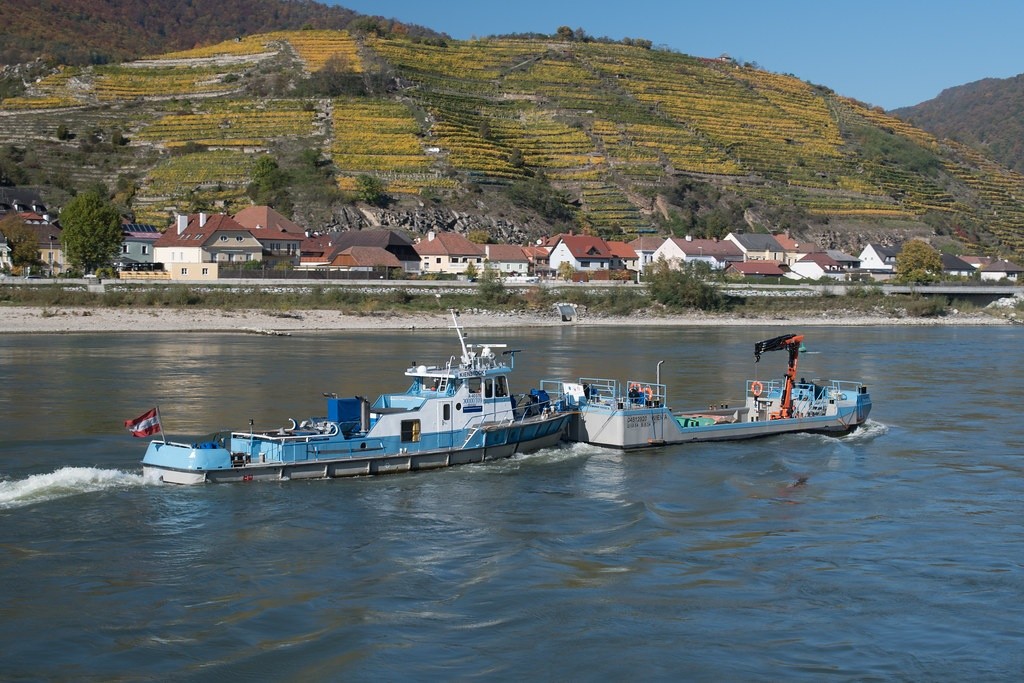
[468,278,478,282]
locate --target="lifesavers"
[751,381,763,396]
[640,388,653,401]
[629,383,641,393]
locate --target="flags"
[125,407,160,438]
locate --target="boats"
[559,334,873,452]
[139,311,574,484]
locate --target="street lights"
[698,246,702,261]
[48,234,54,275]
